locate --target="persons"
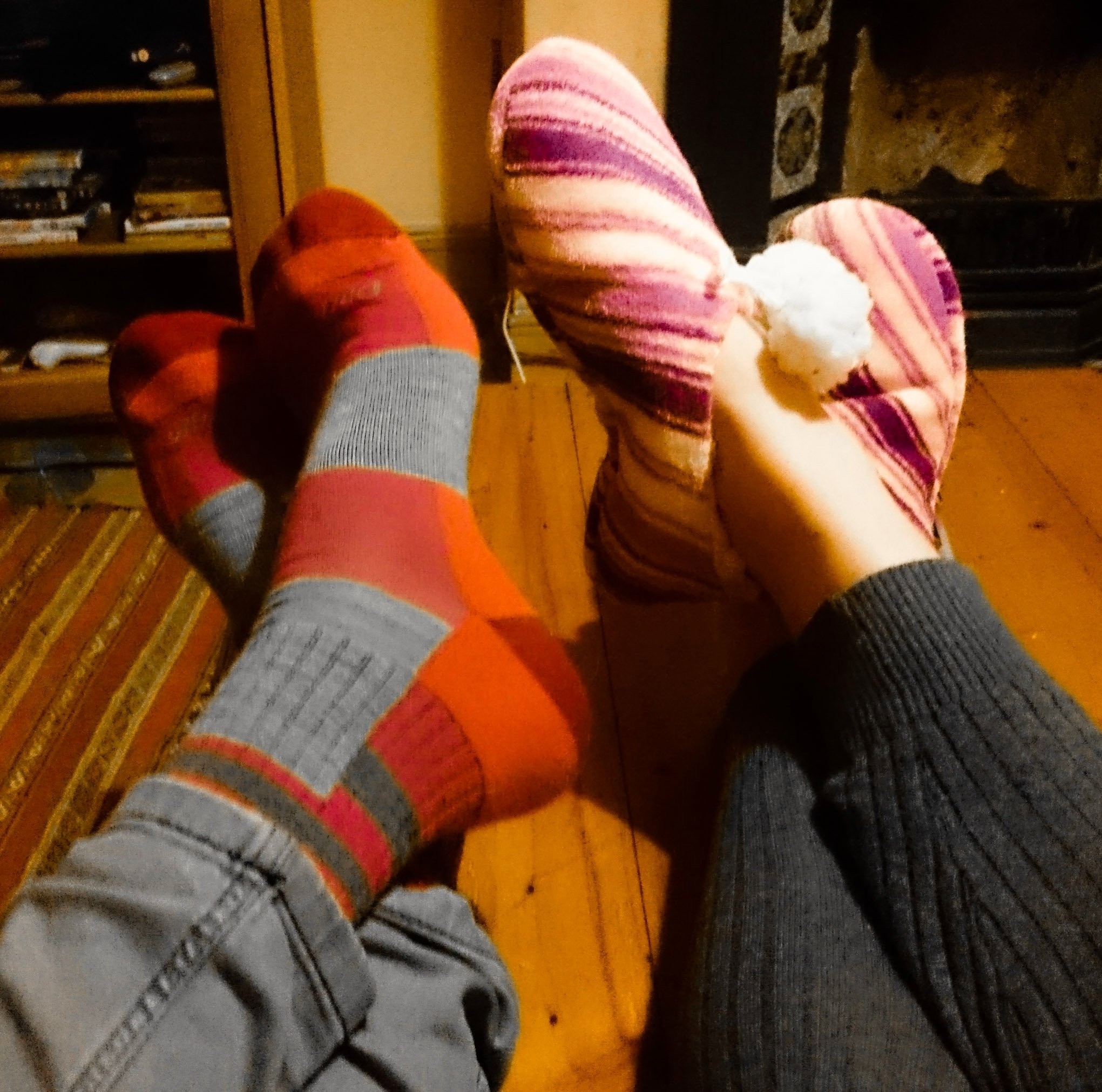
[0,188,594,1092]
[483,34,1102,1092]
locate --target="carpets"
[0,435,258,926]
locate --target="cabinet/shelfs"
[0,0,295,461]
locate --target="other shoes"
[487,34,874,607]
[716,197,968,601]
